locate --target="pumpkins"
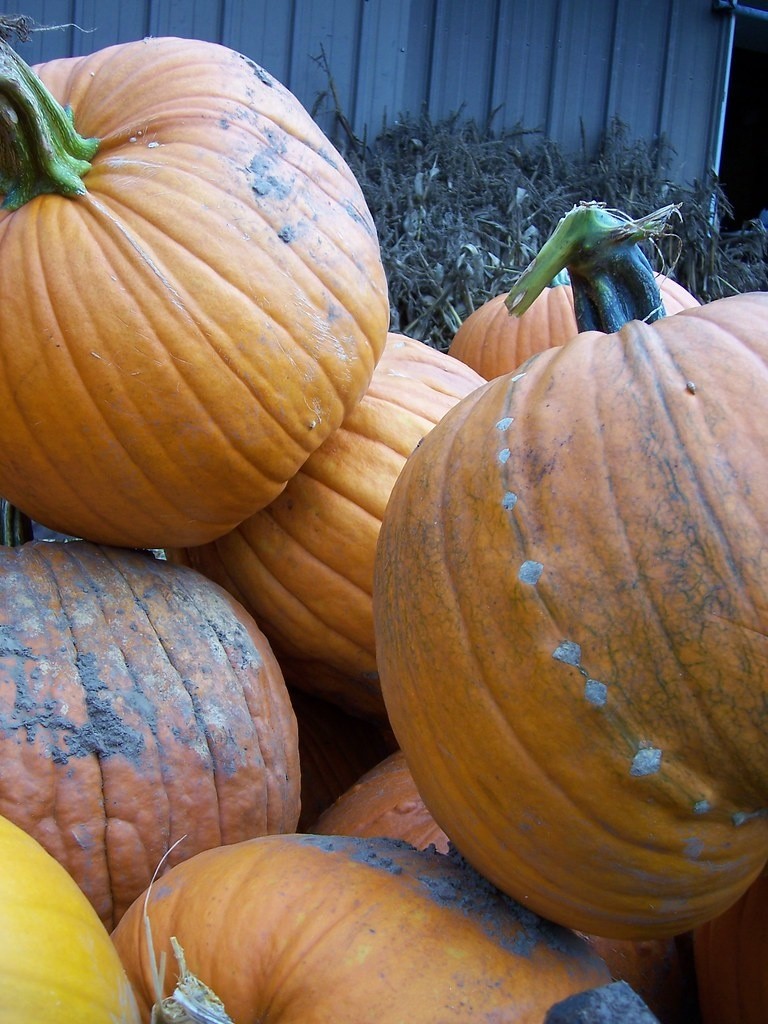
[0,38,768,1024]
